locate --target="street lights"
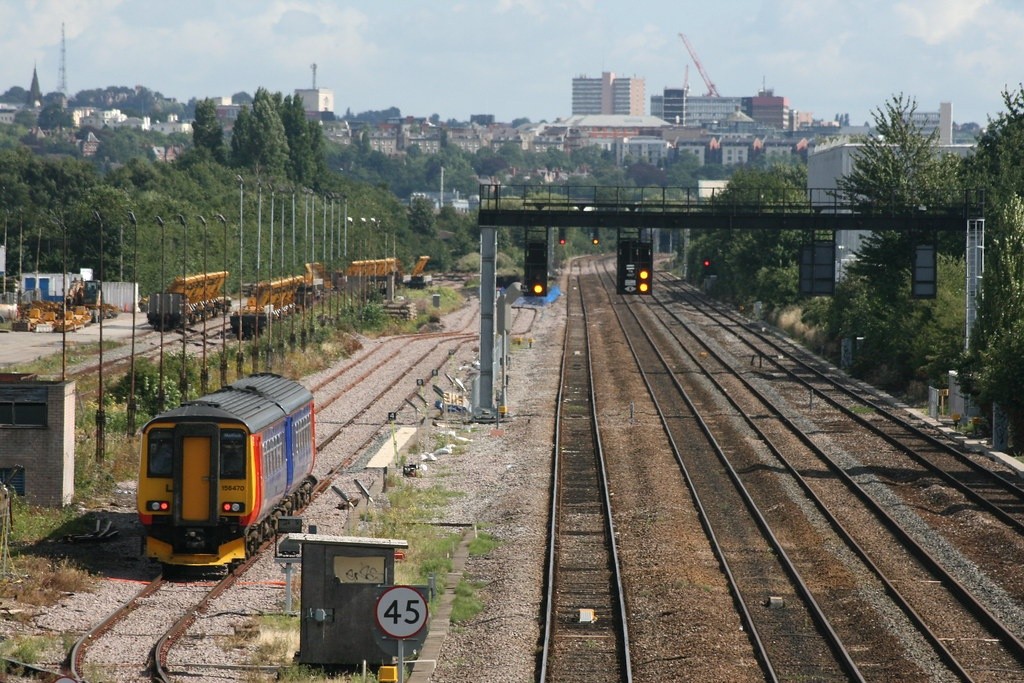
[265,181,276,372]
[217,213,230,387]
[251,180,263,375]
[172,213,191,404]
[279,185,389,361]
[156,215,165,406]
[197,214,214,391]
[92,211,109,462]
[234,173,249,377]
[128,211,141,438]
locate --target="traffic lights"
[558,230,568,248]
[593,233,600,245]
[632,242,655,296]
[703,256,715,278]
[524,243,553,306]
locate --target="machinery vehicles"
[67,275,120,324]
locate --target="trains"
[134,373,318,579]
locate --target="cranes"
[678,32,724,93]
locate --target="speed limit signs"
[375,584,430,639]
